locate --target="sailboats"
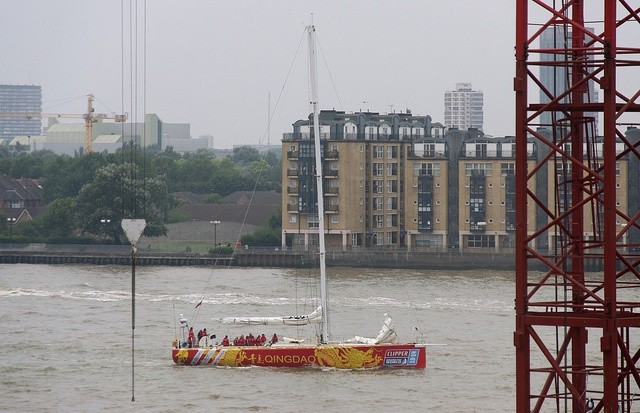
[172,9,427,369]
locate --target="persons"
[223,335,229,346]
[214,241,249,252]
[188,327,195,348]
[289,315,308,319]
[274,245,279,253]
[261,334,268,342]
[248,333,252,346]
[245,336,249,346]
[272,334,278,342]
[251,336,255,346]
[201,328,207,337]
[233,337,238,346]
[197,329,202,341]
[256,335,261,346]
[238,334,244,346]
